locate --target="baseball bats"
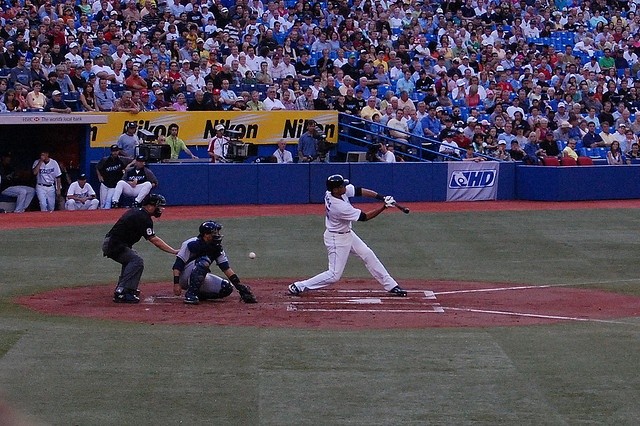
[391,202,410,214]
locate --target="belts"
[38,183,54,187]
[330,231,350,234]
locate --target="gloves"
[383,196,397,208]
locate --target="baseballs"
[249,252,256,259]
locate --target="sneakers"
[288,284,299,296]
[113,286,141,304]
[184,292,199,304]
[390,286,407,297]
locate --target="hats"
[77,174,86,180]
[436,106,443,112]
[498,140,507,145]
[456,79,465,87]
[561,121,572,128]
[52,90,61,95]
[182,59,189,65]
[127,122,136,128]
[213,89,221,95]
[110,11,118,15]
[111,145,119,151]
[467,117,477,124]
[557,102,565,108]
[136,155,145,161]
[249,26,256,30]
[196,38,204,43]
[5,40,14,48]
[155,89,164,95]
[69,42,77,48]
[215,124,224,131]
[221,7,228,12]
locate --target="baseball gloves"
[239,285,258,303]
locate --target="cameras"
[222,130,258,159]
[135,129,172,163]
[312,125,335,156]
[365,143,381,162]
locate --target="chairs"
[340,111,640,165]
[120,194,151,210]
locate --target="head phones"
[124,125,127,130]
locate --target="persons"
[66,174,100,209]
[207,124,230,163]
[1,0,639,112]
[115,123,150,160]
[297,119,320,162]
[165,123,199,159]
[154,134,175,160]
[173,220,256,304]
[273,138,293,163]
[356,112,640,162]
[110,155,159,207]
[97,144,125,207]
[6,161,34,212]
[287,174,408,296]
[32,148,65,212]
[101,193,181,302]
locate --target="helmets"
[141,193,166,218]
[199,221,224,247]
[326,175,350,188]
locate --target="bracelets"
[228,274,239,284]
[375,192,384,200]
[174,275,180,284]
